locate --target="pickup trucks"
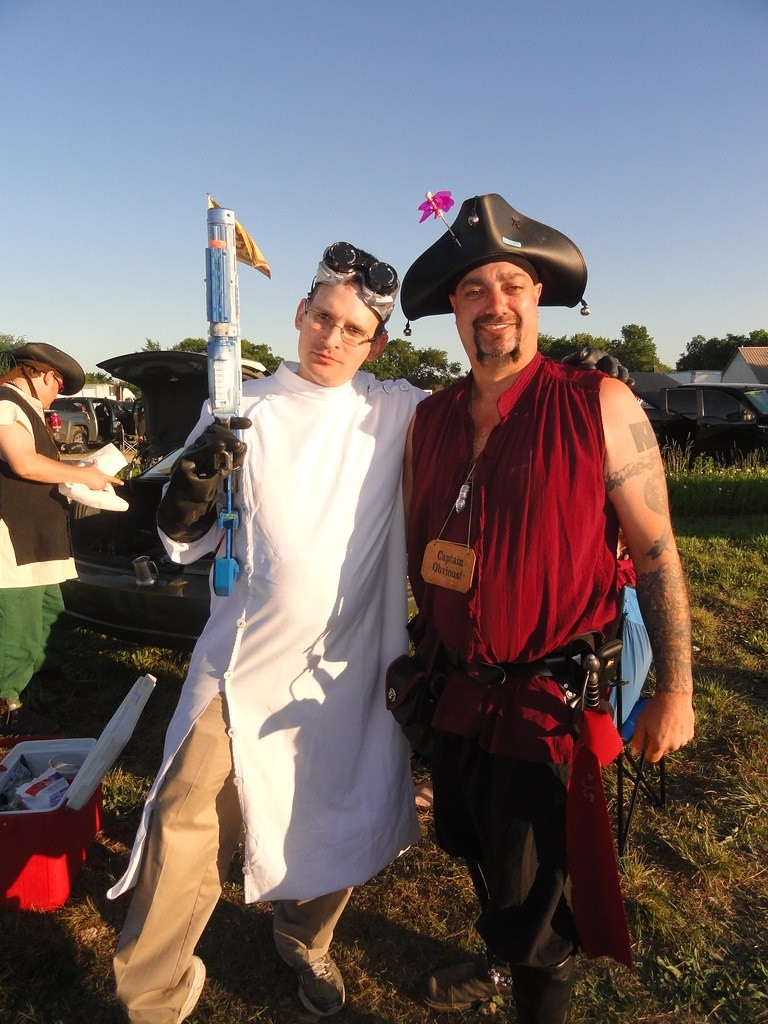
[44,398,134,446]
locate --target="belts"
[441,650,586,685]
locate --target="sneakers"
[176,952,206,1024]
[271,924,346,1016]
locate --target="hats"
[401,193,591,336]
[9,339,86,396]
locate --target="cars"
[629,372,768,468]
[61,350,276,651]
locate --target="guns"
[205,208,248,596]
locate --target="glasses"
[302,297,385,348]
[308,241,400,341]
[43,368,65,393]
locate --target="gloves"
[157,417,252,543]
[561,347,635,387]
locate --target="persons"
[110,243,634,1024]
[401,193,694,1024]
[0,343,124,737]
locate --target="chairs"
[614,612,667,858]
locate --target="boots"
[511,951,576,1023]
[419,912,513,1011]
[0,697,61,735]
[39,668,99,706]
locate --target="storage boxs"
[0,673,158,911]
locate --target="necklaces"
[455,382,474,514]
